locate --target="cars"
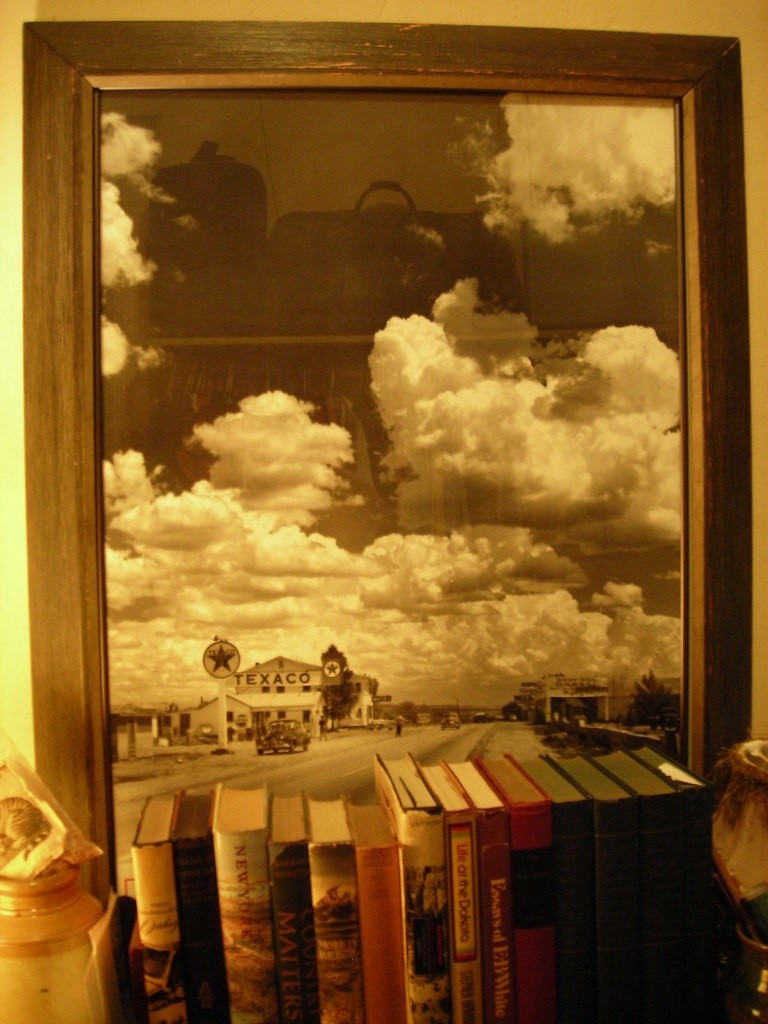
[440,713,462,730]
[256,717,310,754]
[493,711,505,720]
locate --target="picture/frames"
[22,20,754,907]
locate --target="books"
[127,745,720,1024]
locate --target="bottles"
[1,866,105,1024]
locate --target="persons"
[395,716,403,738]
[318,715,327,741]
